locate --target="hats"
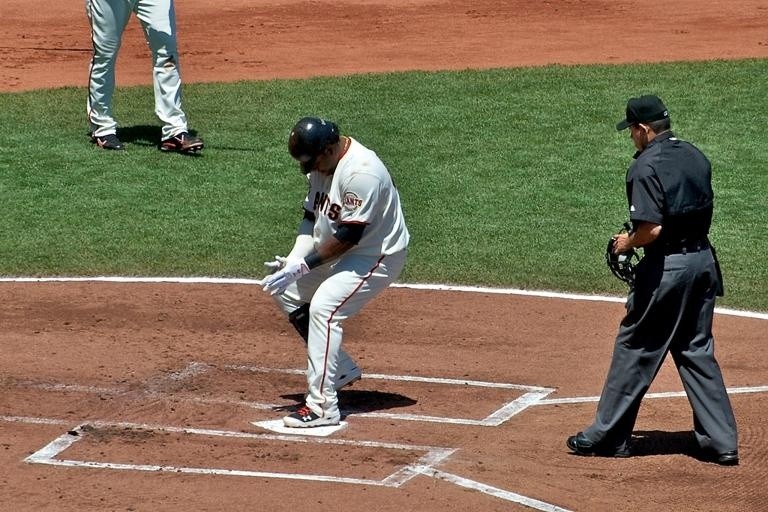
[615,93,671,131]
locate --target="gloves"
[259,254,314,297]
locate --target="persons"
[260,118,411,427]
[567,94,738,464]
[85,0,204,153]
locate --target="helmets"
[288,116,340,176]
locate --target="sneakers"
[686,433,741,468]
[331,365,362,392]
[565,430,632,459]
[283,404,340,430]
[90,131,125,151]
[158,129,206,154]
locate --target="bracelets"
[304,250,325,270]
